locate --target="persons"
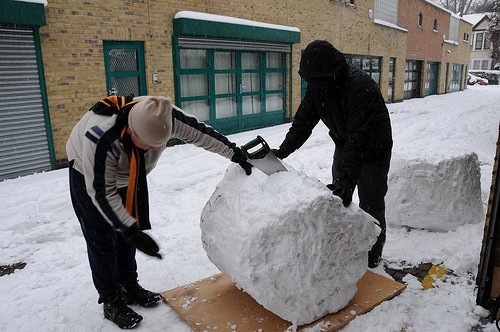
[65,93,253,329]
[269,39,394,269]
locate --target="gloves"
[270,149,287,160]
[231,148,254,176]
[128,225,160,255]
[327,179,353,208]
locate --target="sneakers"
[103,301,143,329]
[121,284,163,307]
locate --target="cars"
[467,73,488,85]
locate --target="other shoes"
[367,250,382,268]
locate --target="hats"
[128,96,172,148]
